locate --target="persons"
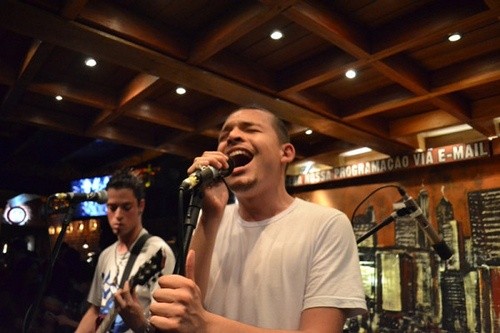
[73,174,176,332]
[5,239,40,318]
[145,107,367,333]
[41,243,89,333]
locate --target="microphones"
[397,186,452,261]
[55,190,109,204]
[180,156,234,191]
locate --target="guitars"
[97,246,168,332]
